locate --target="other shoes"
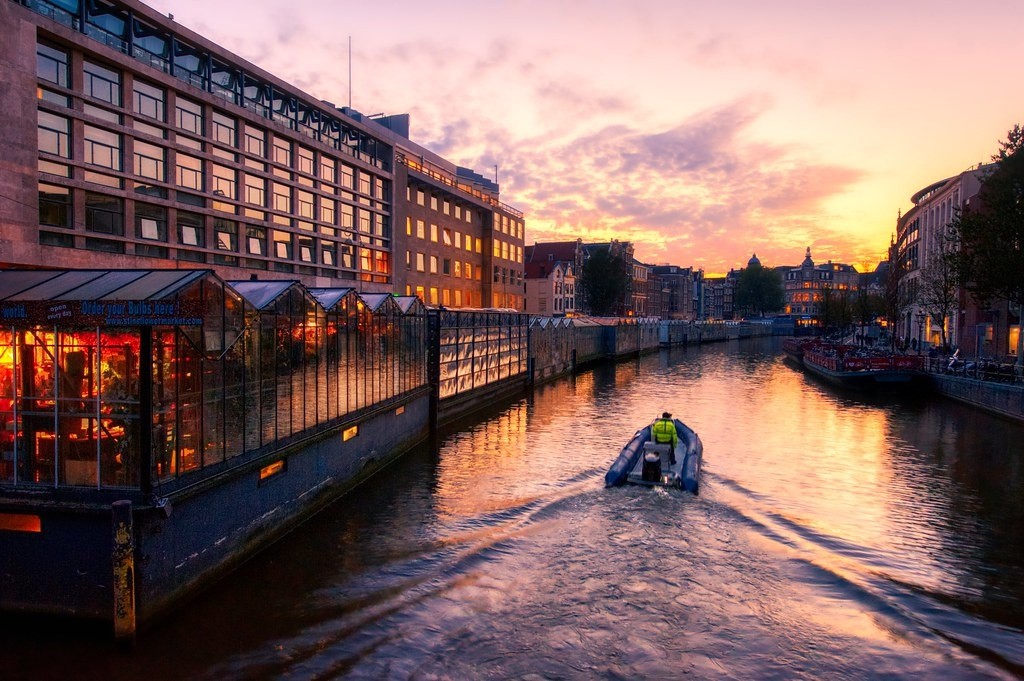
[671,460,677,465]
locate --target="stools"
[644,441,671,471]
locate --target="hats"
[663,412,672,418]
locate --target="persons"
[653,413,678,464]
[857,332,1018,366]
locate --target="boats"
[780,337,931,392]
[604,418,704,496]
